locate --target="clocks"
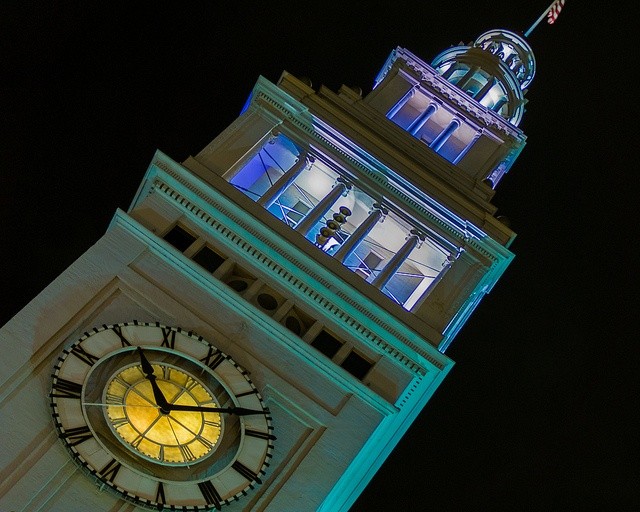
[49,321,277,512]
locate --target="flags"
[548,2,567,22]
